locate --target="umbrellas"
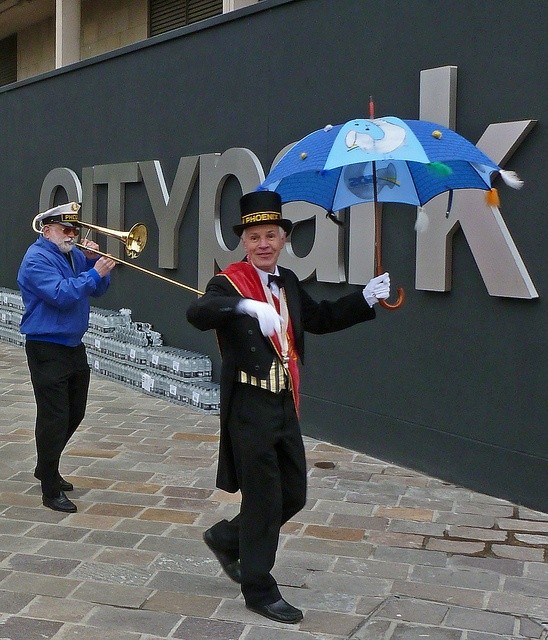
[254,95,524,310]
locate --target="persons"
[186,190,390,625]
[16,201,116,513]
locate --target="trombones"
[33,213,205,296]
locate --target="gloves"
[236,298,284,336]
[362,272,390,307]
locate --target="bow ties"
[267,274,286,288]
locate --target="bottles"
[0,288,27,350]
[83,306,219,418]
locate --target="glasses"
[49,224,80,236]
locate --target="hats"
[35,201,82,227]
[232,191,292,236]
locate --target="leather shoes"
[33,468,73,491]
[43,490,77,512]
[203,519,242,582]
[245,597,303,624]
